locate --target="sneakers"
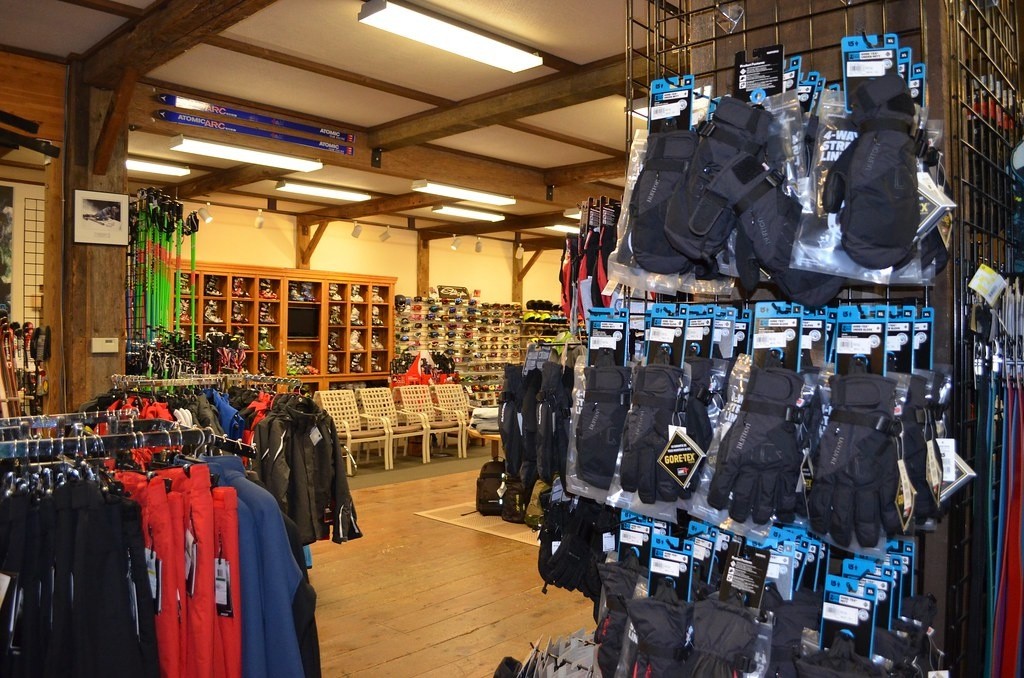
[288,281,316,302]
[394,296,521,407]
[174,275,278,375]
[286,351,319,375]
[327,284,383,373]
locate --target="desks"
[465,427,502,459]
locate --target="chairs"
[433,383,480,459]
[314,385,441,457]
[398,384,463,464]
[318,389,390,477]
[359,387,429,470]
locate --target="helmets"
[524,300,568,348]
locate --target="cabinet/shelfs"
[283,267,326,394]
[327,270,399,390]
[167,259,284,400]
[521,304,589,349]
[393,296,521,408]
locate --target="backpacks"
[524,480,551,533]
[502,481,527,524]
[476,456,505,516]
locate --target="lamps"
[450,233,461,251]
[358,0,544,74]
[411,179,517,207]
[125,157,191,177]
[563,207,581,220]
[475,236,483,253]
[256,208,264,229]
[275,179,372,203]
[351,220,363,239]
[379,225,391,243]
[198,202,213,223]
[431,203,506,223]
[515,243,524,259]
[168,133,324,173]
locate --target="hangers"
[111,373,310,411]
[0,408,260,511]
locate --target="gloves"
[498,72,967,678]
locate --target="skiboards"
[1,302,52,418]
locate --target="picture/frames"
[72,187,129,246]
